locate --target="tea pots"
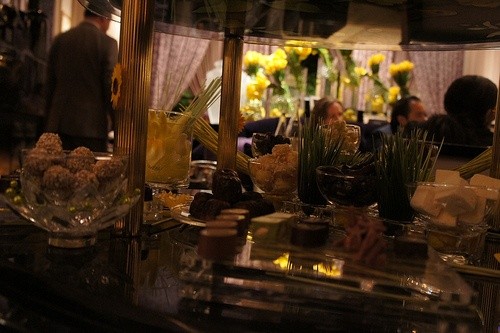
[211,169,242,203]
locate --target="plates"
[171,202,212,226]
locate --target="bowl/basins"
[316,166,384,210]
[1,188,141,246]
[248,158,298,198]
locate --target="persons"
[41,0,119,153]
[308,74,497,164]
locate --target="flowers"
[236,40,415,134]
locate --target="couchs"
[238,118,372,156]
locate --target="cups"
[190,191,275,258]
[143,201,163,224]
[406,184,499,235]
[146,110,194,187]
[325,125,360,155]
